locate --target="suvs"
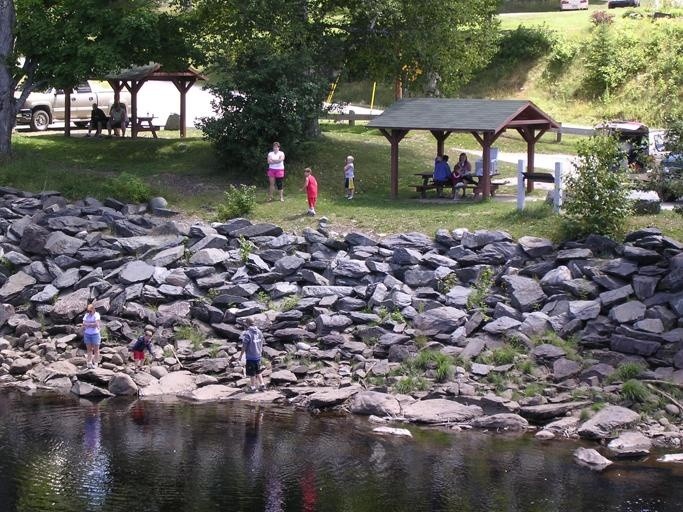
[593,119,682,203]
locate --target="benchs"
[408,180,510,196]
[70,124,160,131]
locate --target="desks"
[136,117,161,141]
[414,171,500,198]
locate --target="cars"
[607,0,640,8]
[558,0,588,10]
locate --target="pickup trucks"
[12,76,131,132]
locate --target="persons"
[266,142,285,202]
[245,409,264,471]
[239,316,267,393]
[131,330,157,374]
[299,168,318,216]
[86,103,128,138]
[344,155,355,200]
[84,403,100,466]
[82,304,101,365]
[433,153,471,199]
